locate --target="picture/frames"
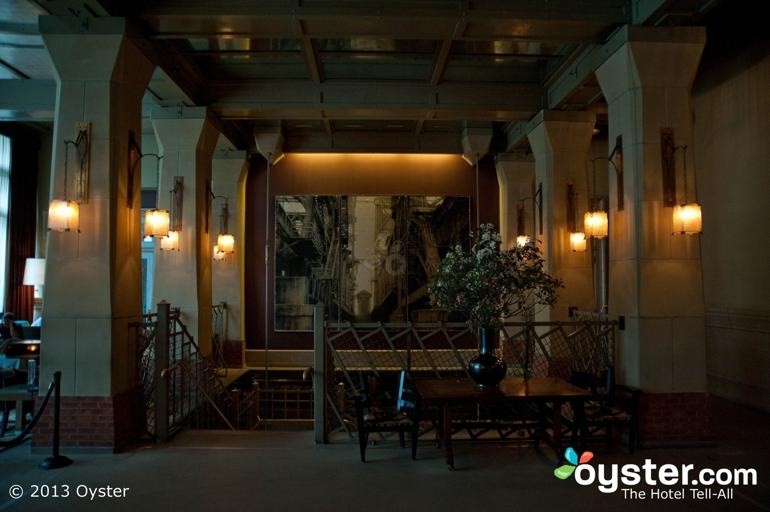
[273,192,473,334]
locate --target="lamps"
[45,119,94,235]
[22,258,47,326]
[516,183,542,249]
[659,127,707,243]
[142,177,234,262]
[565,133,624,253]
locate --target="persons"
[0,312,24,369]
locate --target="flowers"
[423,219,565,324]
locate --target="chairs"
[347,365,644,472]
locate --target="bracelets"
[10,327,14,329]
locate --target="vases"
[466,321,509,383]
